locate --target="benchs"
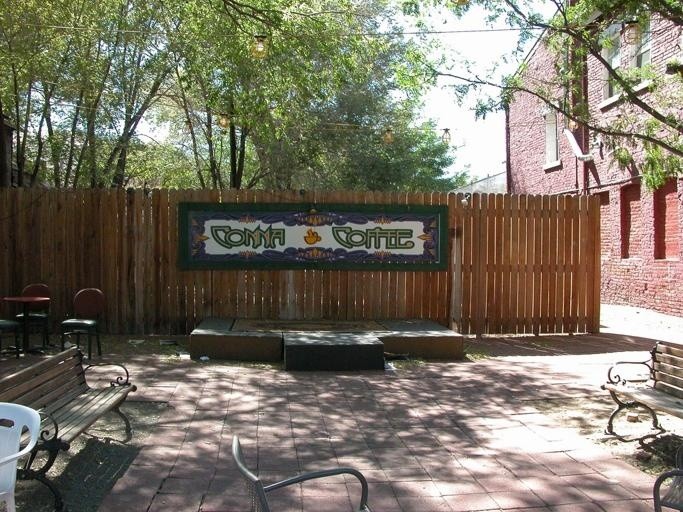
[0,346,137,512]
[600,341,683,435]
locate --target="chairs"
[0,283,105,360]
[230,434,372,511]
[0,402,41,512]
